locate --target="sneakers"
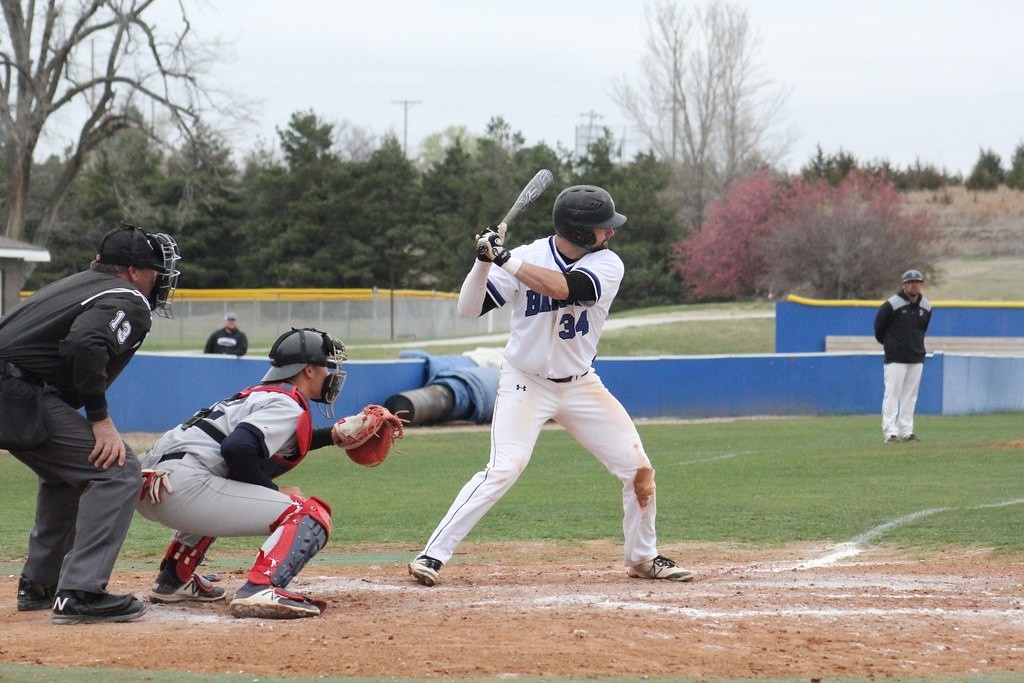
[50,592,147,624]
[628,556,693,581]
[230,588,320,619]
[149,576,226,602]
[410,553,442,585]
[17,578,57,610]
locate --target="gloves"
[140,469,173,504]
[477,232,523,276]
[475,223,507,244]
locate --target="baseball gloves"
[331,404,404,467]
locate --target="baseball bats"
[476,169,554,255]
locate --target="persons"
[201,312,249,356]
[0,222,182,626]
[409,185,693,587]
[134,326,403,619]
[873,269,933,444]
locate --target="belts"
[547,371,588,382]
[157,452,186,463]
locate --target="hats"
[95,221,162,271]
[226,313,235,320]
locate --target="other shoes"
[900,434,919,441]
[885,436,899,443]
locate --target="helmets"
[552,185,627,248]
[261,331,334,382]
[902,270,923,284]
